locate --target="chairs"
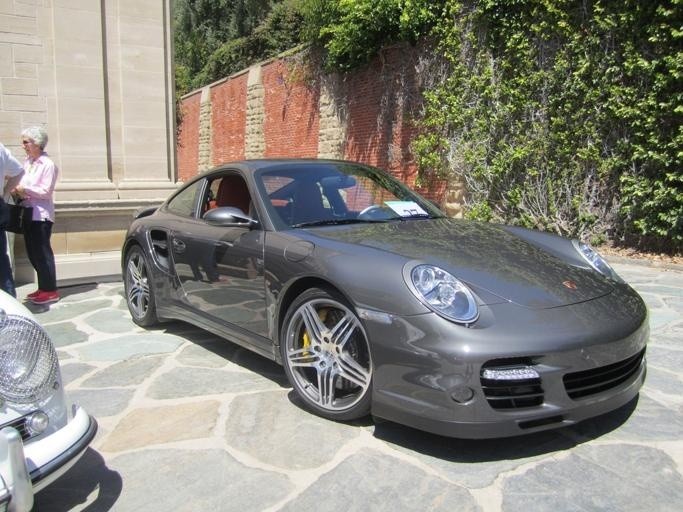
[290,181,325,225]
[217,176,250,215]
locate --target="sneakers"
[28,290,60,304]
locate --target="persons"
[8,125,59,304]
[0,141,26,298]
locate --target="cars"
[0,287,100,511]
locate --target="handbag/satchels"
[1,201,33,234]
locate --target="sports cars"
[120,157,650,442]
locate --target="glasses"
[23,140,29,144]
